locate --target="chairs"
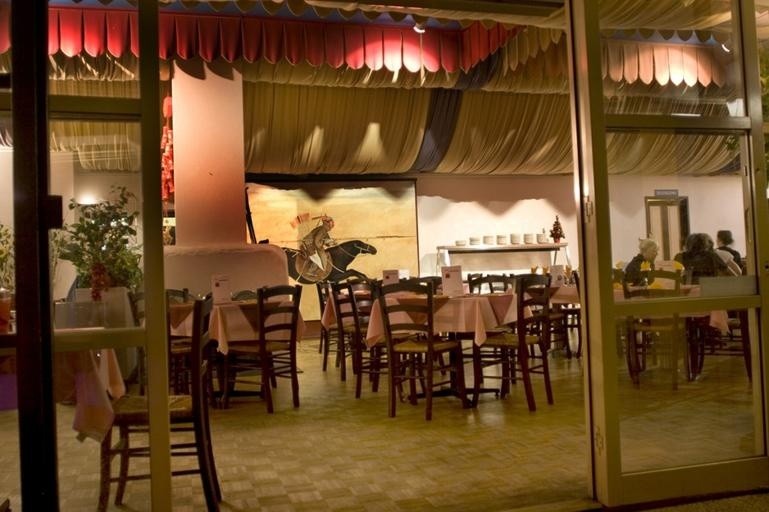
[229,286,279,391]
[125,287,190,396]
[220,283,304,415]
[97,291,225,512]
[621,267,691,391]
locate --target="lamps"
[720,38,731,54]
[411,20,427,35]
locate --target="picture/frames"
[243,176,422,325]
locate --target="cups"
[455,234,545,246]
[0,289,10,335]
[531,264,572,274]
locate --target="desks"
[436,241,573,268]
[168,301,310,356]
[612,283,730,338]
[0,325,128,445]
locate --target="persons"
[680,233,739,332]
[622,239,658,343]
[714,230,743,268]
[296,215,335,284]
[710,249,742,334]
[674,238,685,267]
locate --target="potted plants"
[50,183,146,302]
